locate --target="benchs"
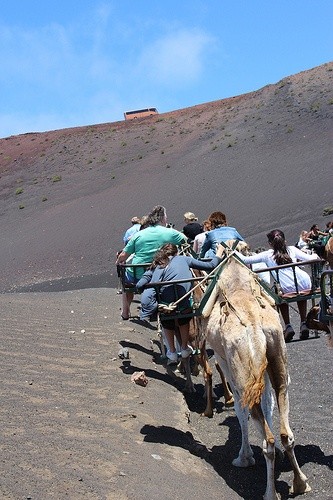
[118,258,216,288]
[141,257,323,320]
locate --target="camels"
[187,221,312,500]
[325,236,333,299]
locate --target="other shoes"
[121,311,129,320]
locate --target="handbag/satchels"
[306,305,330,334]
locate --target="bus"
[124,107,159,121]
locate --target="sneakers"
[299,321,309,339]
[283,324,295,341]
[166,349,177,360]
[181,345,194,358]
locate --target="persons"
[135,243,226,361]
[235,229,319,342]
[114,205,333,320]
